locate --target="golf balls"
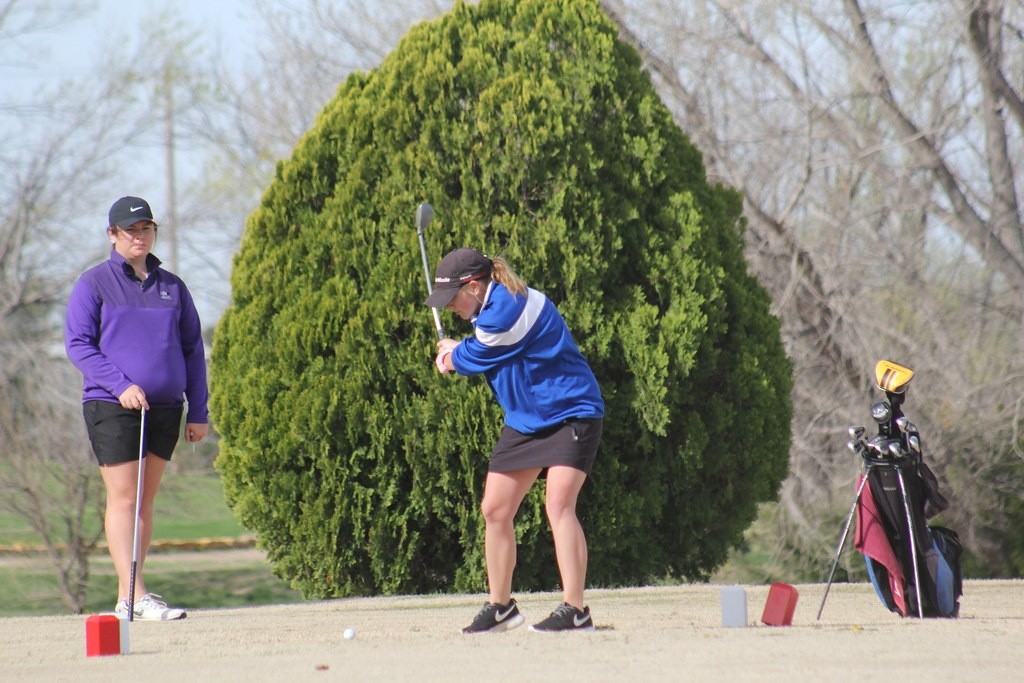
[343,628,355,639]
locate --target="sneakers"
[529,602,595,632]
[458,599,525,636]
[119,592,187,620]
[114,601,122,612]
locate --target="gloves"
[436,349,452,374]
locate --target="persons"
[66,196,208,622]
[424,247,605,634]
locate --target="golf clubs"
[845,401,921,461]
[125,405,147,620]
[414,203,455,379]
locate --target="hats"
[423,248,494,307]
[108,196,158,229]
